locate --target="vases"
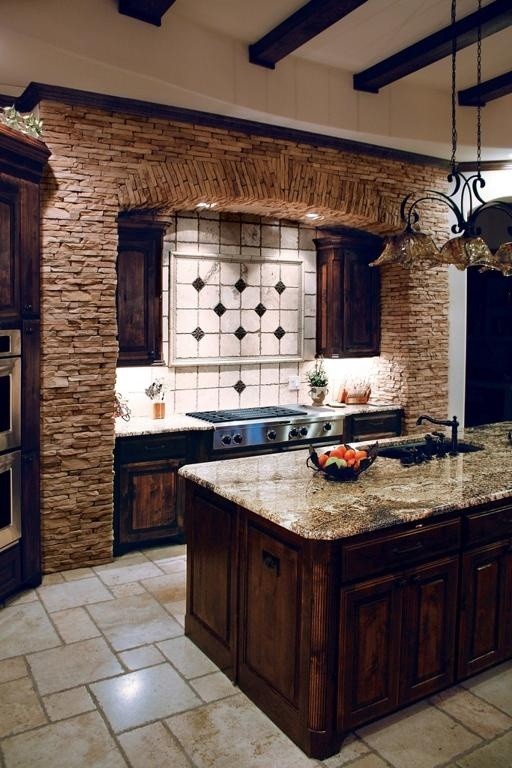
[152,401,165,418]
[306,386,330,407]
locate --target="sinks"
[355,442,414,459]
[402,434,486,456]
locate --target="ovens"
[0,327,26,557]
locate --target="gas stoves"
[185,405,346,449]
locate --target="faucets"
[416,414,459,453]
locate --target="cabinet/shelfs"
[183,480,318,757]
[317,510,462,760]
[461,499,512,685]
[115,220,173,366]
[1,123,56,316]
[113,432,206,553]
[311,234,382,359]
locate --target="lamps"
[365,1,512,276]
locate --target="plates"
[328,402,346,408]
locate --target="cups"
[153,400,166,419]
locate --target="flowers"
[145,377,169,403]
[303,351,331,386]
